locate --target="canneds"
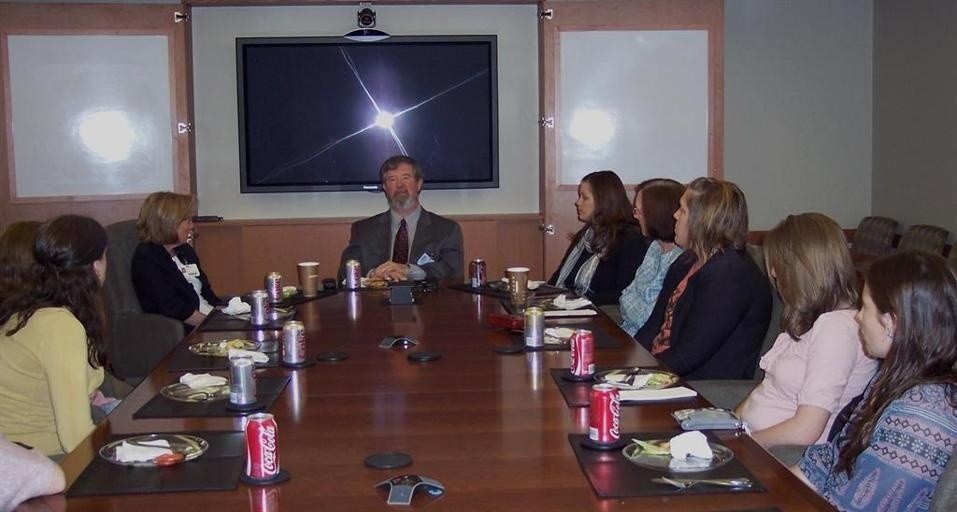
[470,259,488,288]
[589,383,622,445]
[284,321,307,365]
[571,382,589,432]
[524,351,545,390]
[246,485,281,512]
[570,331,597,375]
[470,294,484,319]
[265,270,284,302]
[230,353,257,404]
[250,290,271,324]
[589,456,618,511]
[525,308,546,347]
[346,293,363,319]
[245,414,280,480]
[285,368,308,410]
[345,259,360,290]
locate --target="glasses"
[633,207,643,215]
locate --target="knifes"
[652,477,747,484]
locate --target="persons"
[0,222,135,401]
[618,178,688,338]
[130,192,227,336]
[633,177,774,380]
[0,215,108,464]
[733,212,883,452]
[337,156,465,286]
[0,438,67,512]
[548,170,645,304]
[797,250,957,512]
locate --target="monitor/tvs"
[235,28,499,193]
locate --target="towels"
[343,276,367,287]
[543,327,576,343]
[604,373,652,389]
[179,373,226,389]
[62,278,838,511]
[220,297,251,315]
[551,294,592,309]
[502,277,544,290]
[617,386,698,401]
[670,406,742,430]
[544,309,597,317]
[668,430,712,459]
[229,349,269,363]
[116,439,171,462]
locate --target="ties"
[651,265,697,358]
[392,218,409,264]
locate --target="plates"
[98,433,201,466]
[621,438,732,472]
[187,340,257,357]
[159,378,226,403]
[593,367,675,390]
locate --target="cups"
[506,267,530,306]
[297,262,319,298]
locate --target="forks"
[662,475,751,490]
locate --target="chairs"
[96,218,186,384]
[686,248,793,407]
[893,225,951,257]
[851,215,899,254]
[598,298,623,325]
[768,439,957,512]
